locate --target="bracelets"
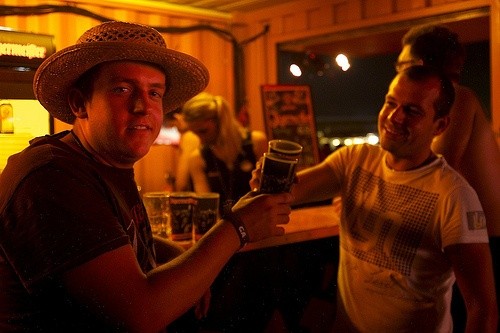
[222,208,250,249]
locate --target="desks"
[169,200,339,255]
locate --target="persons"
[249,66,500,333]
[167,92,269,218]
[396,23,500,333]
[0,22,294,333]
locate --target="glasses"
[394,60,415,72]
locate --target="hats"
[33,22,209,126]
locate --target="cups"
[192,192,220,244]
[143,192,170,240]
[169,192,192,242]
[259,153,298,196]
[267,139,303,159]
